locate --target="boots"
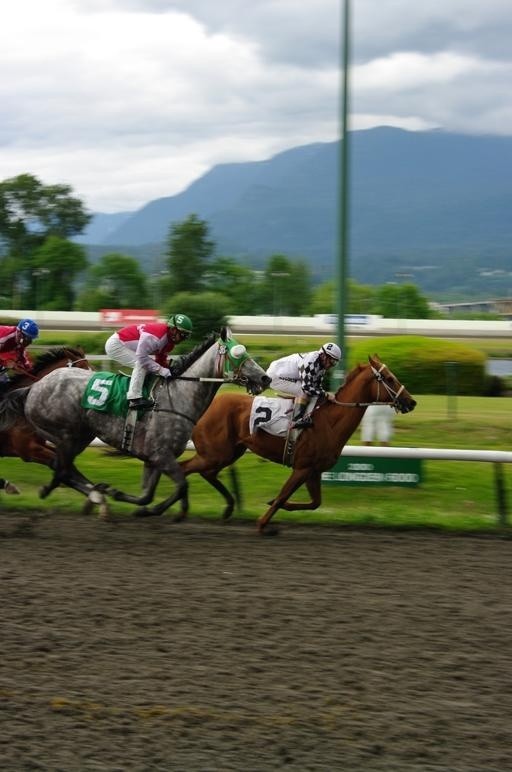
[289,403,312,429]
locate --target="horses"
[169,353,416,538]
[1,324,272,522]
[0,343,98,523]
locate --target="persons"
[0,318,41,384]
[265,341,343,429]
[103,313,195,411]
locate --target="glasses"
[177,330,191,339]
[329,357,338,366]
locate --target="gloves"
[159,366,171,379]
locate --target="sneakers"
[129,398,154,410]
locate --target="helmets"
[16,319,38,338]
[167,314,192,333]
[318,343,341,360]
